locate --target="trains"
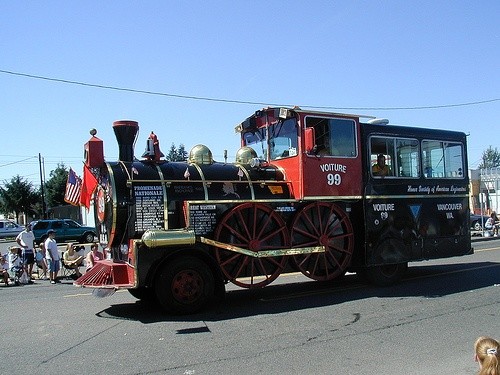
[62,105,475,312]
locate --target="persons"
[0,230,106,287]
[373,154,393,176]
[471,335,500,375]
[45,229,61,284]
[15,222,37,283]
[485,211,500,238]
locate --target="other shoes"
[76,273,82,277]
[64,265,70,269]
[493,234,500,238]
[50,280,61,284]
[29,276,35,280]
[42,276,48,280]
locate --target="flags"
[80,165,98,210]
[64,169,82,206]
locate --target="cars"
[470,212,491,231]
[0,220,24,239]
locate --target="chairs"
[1,273,12,286]
[481,224,494,238]
[35,255,51,280]
[60,252,85,279]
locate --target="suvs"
[27,218,97,244]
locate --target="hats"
[9,245,21,250]
[47,229,56,234]
